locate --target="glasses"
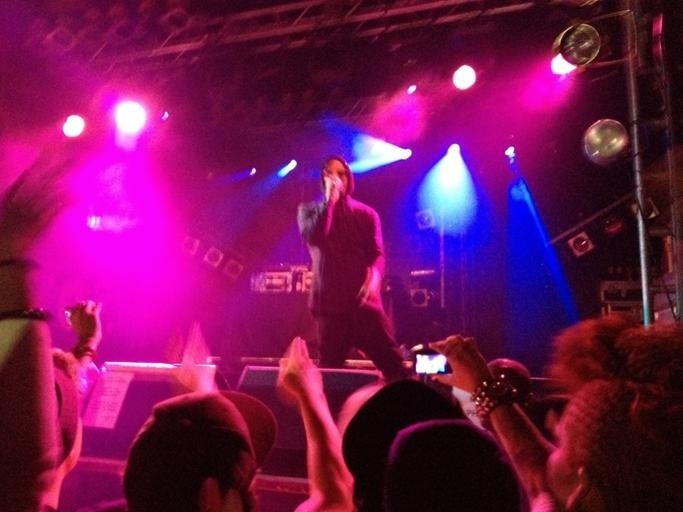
[323,169,345,176]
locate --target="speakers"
[76,361,232,462]
[236,366,386,478]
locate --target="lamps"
[584,118,632,167]
[551,23,601,66]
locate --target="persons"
[292,154,408,380]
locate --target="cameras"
[64,305,76,326]
[413,350,449,376]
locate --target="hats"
[487,357,531,381]
[341,377,532,510]
[122,390,278,509]
